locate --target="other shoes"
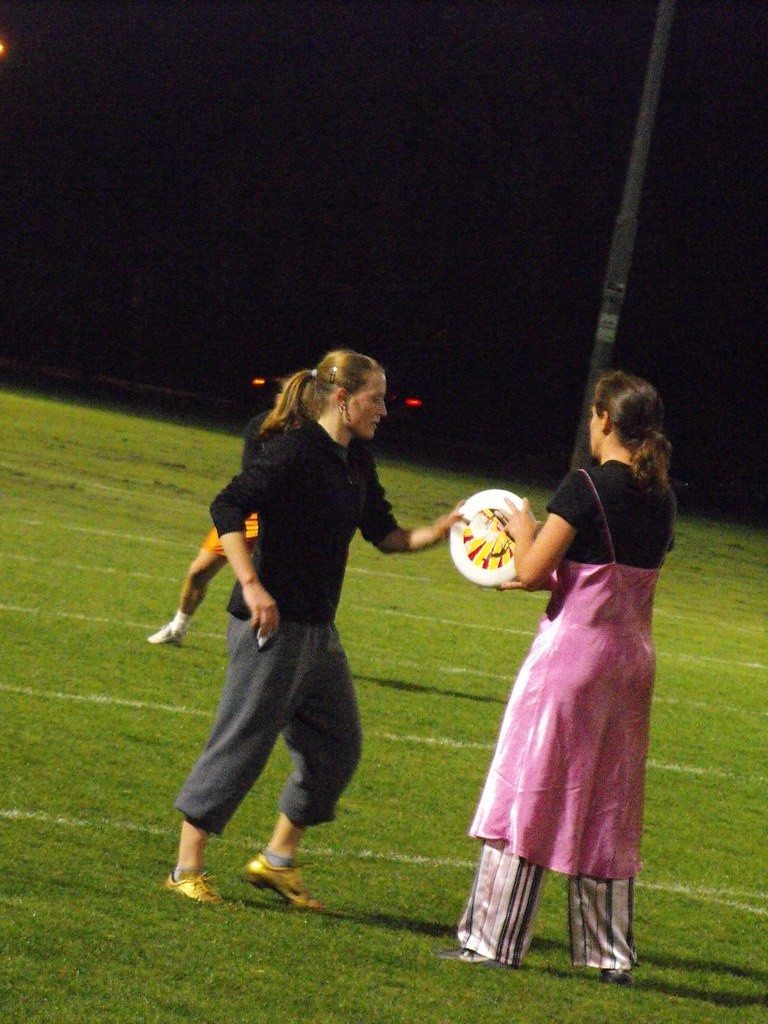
[601,968,635,987]
[438,948,512,967]
[148,625,183,644]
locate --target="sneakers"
[239,853,326,909]
[165,872,238,911]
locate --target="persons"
[148,380,286,646]
[164,349,470,909]
[440,373,676,983]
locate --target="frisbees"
[448,487,536,586]
